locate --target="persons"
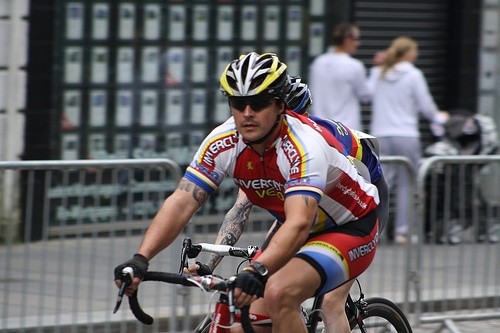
[308,22,386,132]
[185,72,389,333]
[371,36,451,244]
[115,50,379,333]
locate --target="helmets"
[219,51,288,99]
[282,74,312,115]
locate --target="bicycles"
[111,236,414,333]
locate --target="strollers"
[417,110,500,246]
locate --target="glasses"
[229,95,278,113]
[350,35,360,42]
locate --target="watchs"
[250,261,269,281]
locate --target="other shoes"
[394,232,418,245]
